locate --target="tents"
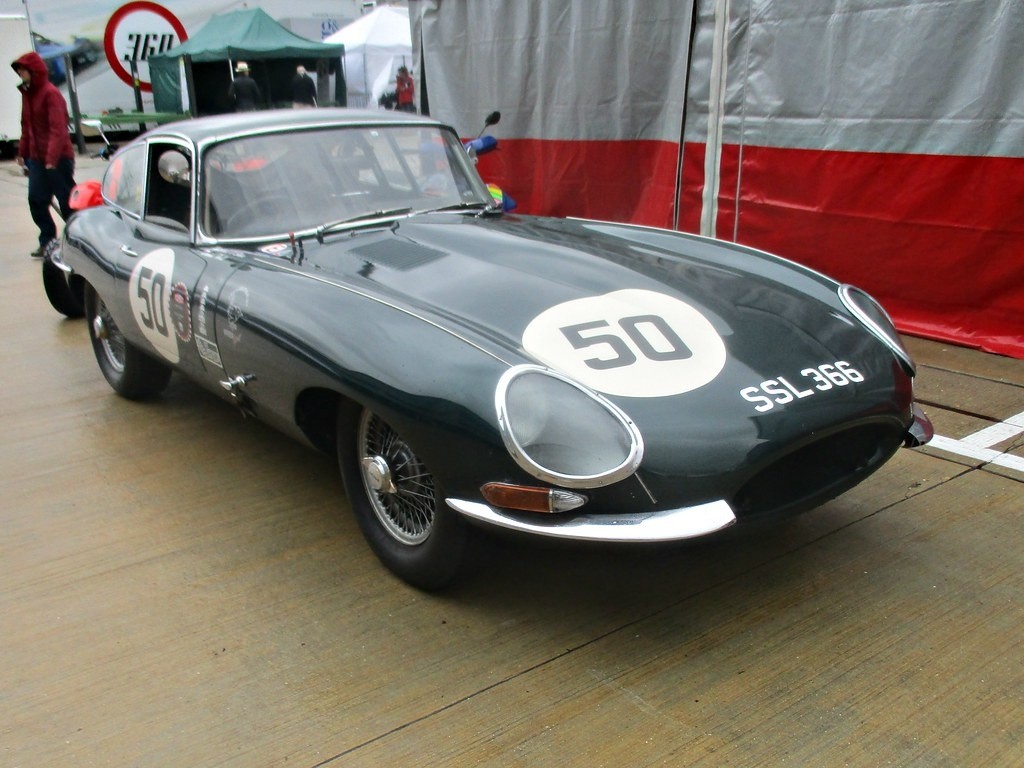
[147,7,348,127]
[321,6,414,111]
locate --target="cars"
[44,109,935,595]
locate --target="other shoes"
[31,248,43,257]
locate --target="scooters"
[336,111,519,214]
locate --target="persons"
[396,67,417,112]
[228,61,260,112]
[291,66,316,110]
[10,51,77,259]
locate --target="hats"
[235,62,250,72]
